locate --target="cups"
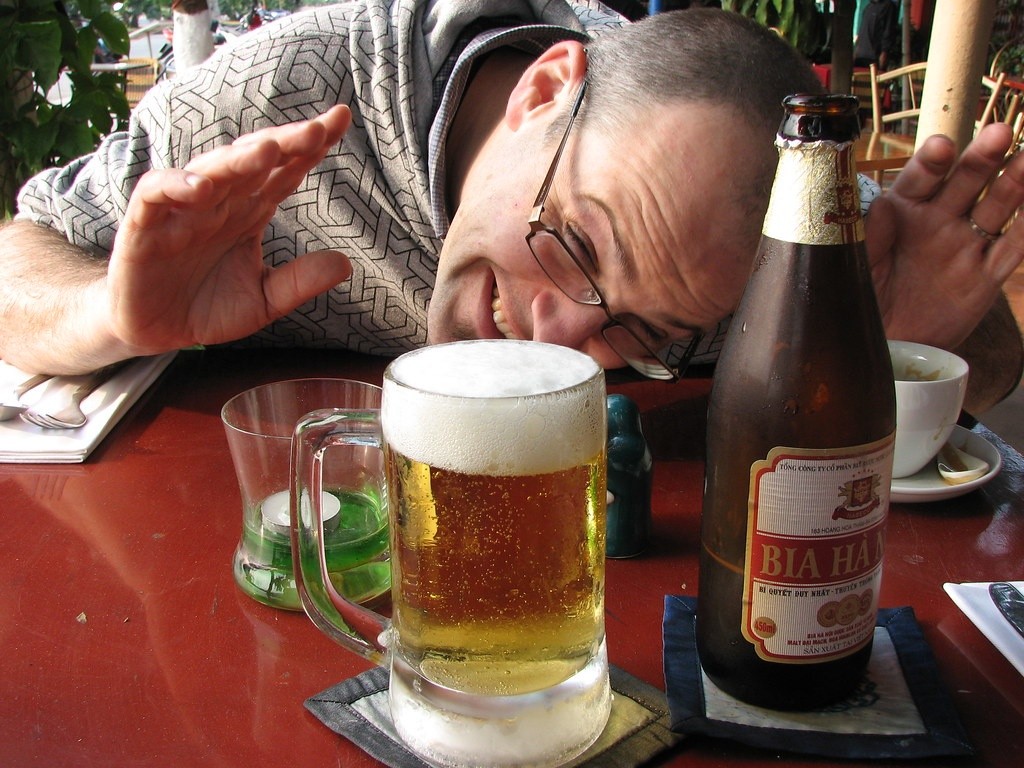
[884,340,969,479]
[220,379,391,613]
[290,340,611,768]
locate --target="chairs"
[123,58,166,109]
[867,33,1024,191]
[852,72,887,131]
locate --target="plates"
[890,425,1001,502]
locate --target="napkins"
[0,350,179,463]
[943,581,1024,679]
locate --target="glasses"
[526,69,704,384]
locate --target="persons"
[854,1,897,69]
[94,30,122,79]
[0,0,1024,449]
[247,6,262,31]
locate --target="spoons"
[936,442,990,484]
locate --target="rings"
[968,215,1001,241]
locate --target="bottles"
[696,97,895,714]
[604,394,650,559]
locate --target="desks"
[0,346,1024,767]
[990,76,1024,91]
[853,133,916,170]
[87,63,151,97]
[821,64,891,110]
